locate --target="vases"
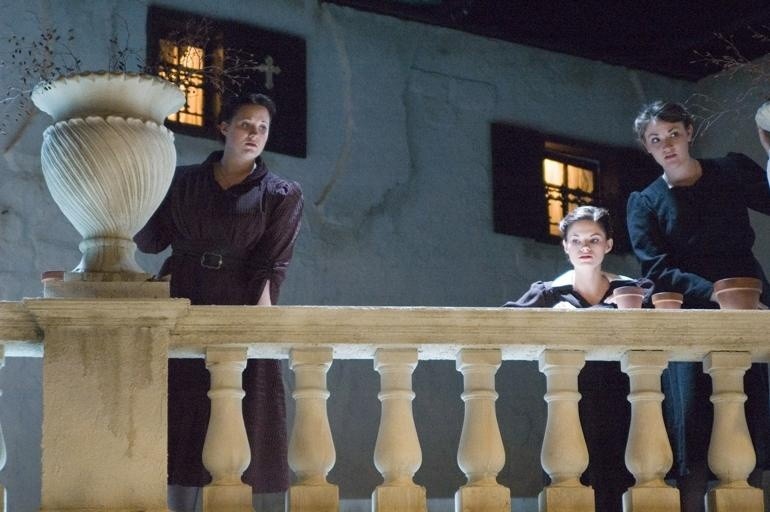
[32,73,188,274]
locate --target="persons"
[626,101,770,512]
[503,206,655,512]
[130,91,303,512]
